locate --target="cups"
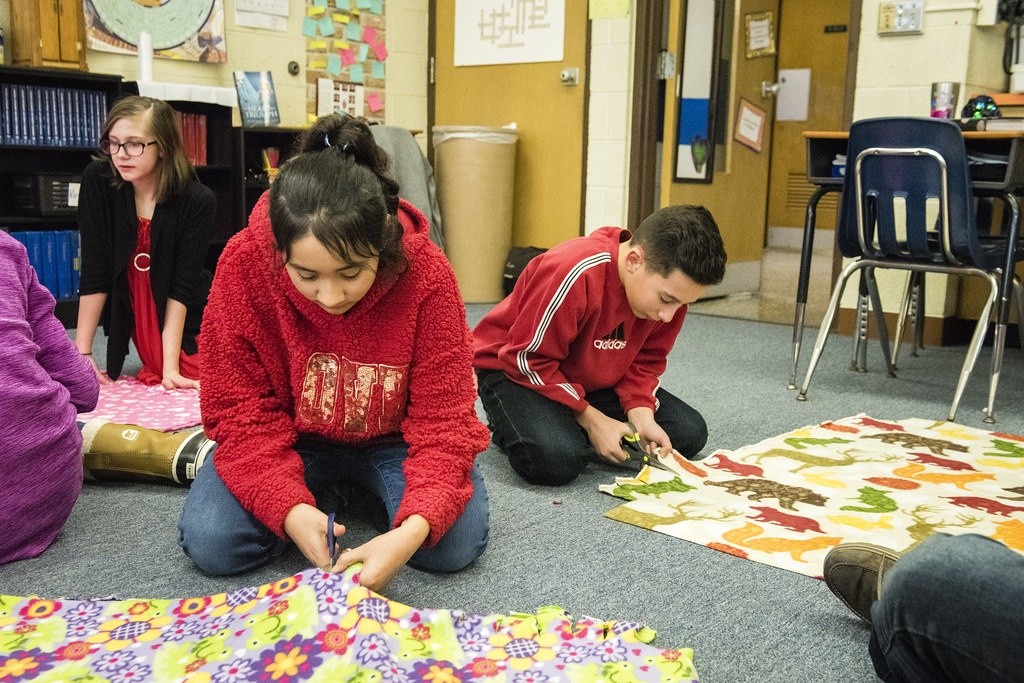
[932,82,961,120]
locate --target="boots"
[79,417,220,489]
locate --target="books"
[0,84,107,147]
[947,93,1024,131]
[176,112,207,166]
[8,230,81,299]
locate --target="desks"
[786,129,1024,424]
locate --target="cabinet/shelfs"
[0,65,313,329]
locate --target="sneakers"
[823,542,904,629]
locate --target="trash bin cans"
[432,125,519,304]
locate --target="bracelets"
[82,353,93,355]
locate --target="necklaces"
[138,216,152,240]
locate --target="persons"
[822,532,1024,683]
[74,97,221,392]
[171,114,492,593]
[474,204,729,485]
[0,229,186,568]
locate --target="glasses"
[98,139,156,157]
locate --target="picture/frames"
[733,96,768,153]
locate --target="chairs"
[796,116,1024,421]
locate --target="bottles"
[1009,64,1024,93]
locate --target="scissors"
[327,513,337,572]
[618,421,680,475]
